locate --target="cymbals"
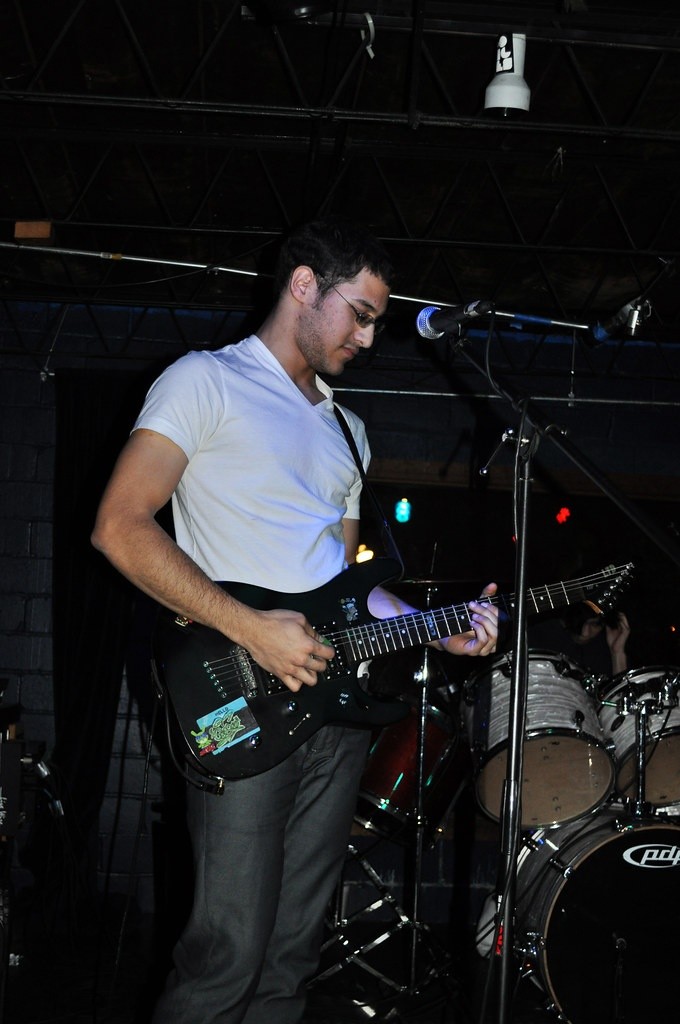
[395,576,477,588]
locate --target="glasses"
[313,268,386,337]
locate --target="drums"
[460,644,620,831]
[590,663,680,808]
[358,697,468,852]
[505,807,680,1024]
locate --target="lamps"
[483,33,531,122]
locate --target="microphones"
[586,294,651,346]
[417,300,497,340]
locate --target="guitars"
[116,556,648,795]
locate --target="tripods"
[306,583,463,996]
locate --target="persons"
[87,200,499,1024]
[487,599,633,687]
